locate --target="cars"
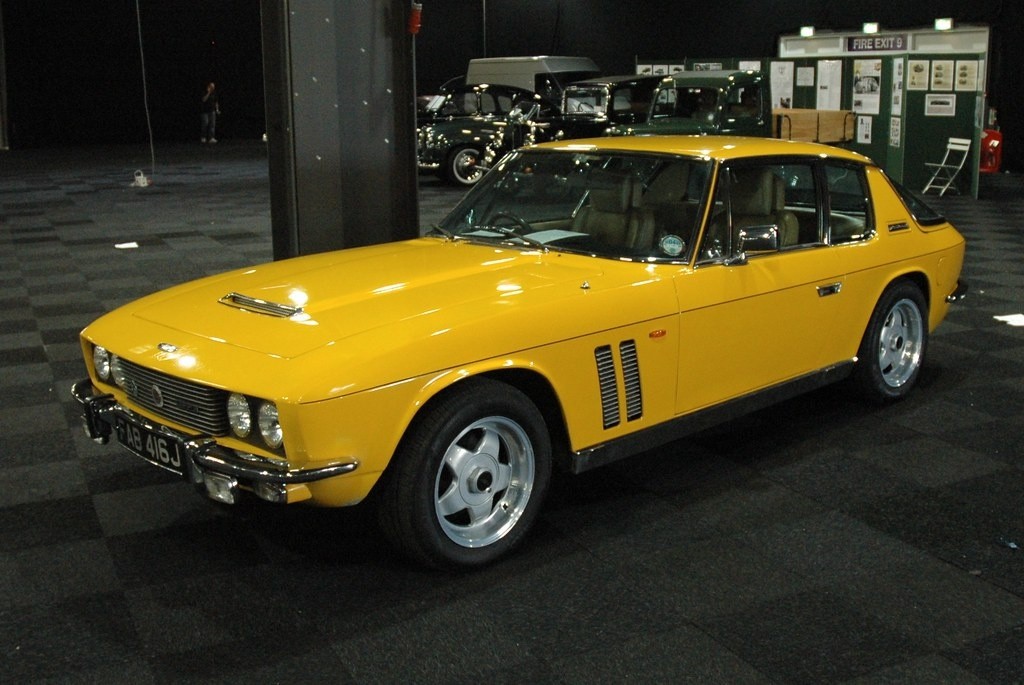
[418,71,671,207]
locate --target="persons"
[201,82,220,143]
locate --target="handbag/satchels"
[133,170,148,187]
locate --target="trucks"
[603,67,884,205]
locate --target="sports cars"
[68,133,969,575]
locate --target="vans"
[461,54,601,116]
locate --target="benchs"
[785,207,865,244]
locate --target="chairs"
[921,137,971,196]
[706,168,800,255]
[569,168,655,253]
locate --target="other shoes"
[201,138,206,142]
[209,138,217,143]
